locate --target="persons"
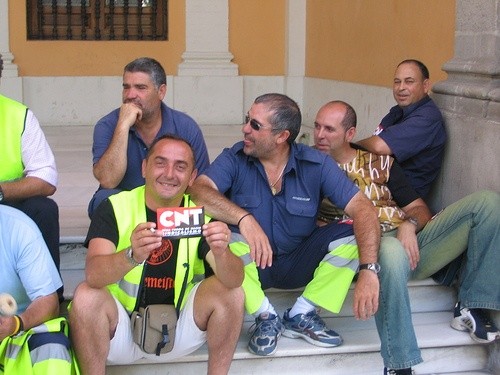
[67,135,245,375]
[0,201,81,375]
[354,61,447,204]
[88,57,210,221]
[0,54,64,306]
[310,101,500,375]
[186,93,381,357]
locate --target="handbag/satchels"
[130,303,178,356]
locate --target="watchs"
[126,245,143,267]
[359,262,381,275]
[0,188,4,203]
[406,216,421,229]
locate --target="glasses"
[246,115,282,130]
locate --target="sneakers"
[281,307,344,348]
[384,367,415,375]
[450,301,500,344]
[247,311,282,356]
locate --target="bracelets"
[9,315,23,338]
[236,212,253,230]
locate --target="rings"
[225,233,228,241]
